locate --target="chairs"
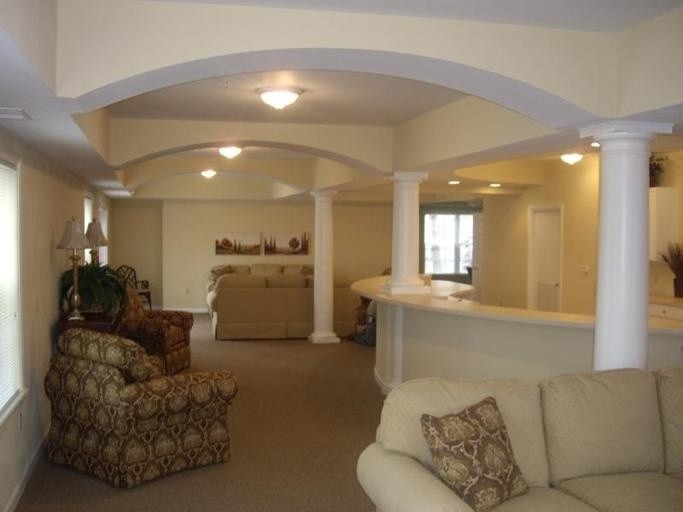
[108,288,193,376]
[43,328,239,488]
[116,264,153,310]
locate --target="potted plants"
[649,155,668,187]
[61,263,127,323]
[662,242,683,297]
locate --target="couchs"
[205,264,363,340]
[356,363,683,512]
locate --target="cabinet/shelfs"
[56,313,111,337]
[648,186,679,262]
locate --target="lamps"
[198,85,306,178]
[56,215,91,321]
[85,218,109,263]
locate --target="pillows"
[420,396,529,512]
